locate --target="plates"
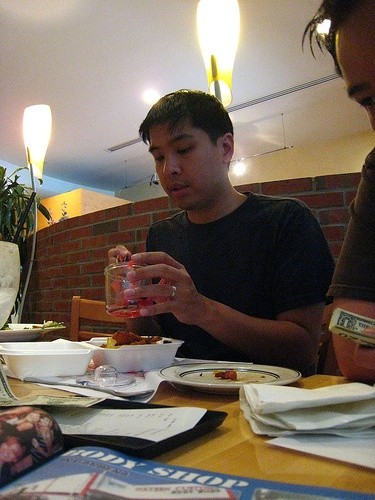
[158,363,302,393]
[0,323,66,342]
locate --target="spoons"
[23,378,155,400]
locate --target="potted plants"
[0,164,52,329]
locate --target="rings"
[170,285,177,297]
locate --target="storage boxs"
[329,308,375,347]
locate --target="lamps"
[22,104,53,186]
[196,0,241,108]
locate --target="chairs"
[70,296,133,342]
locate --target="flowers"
[48,201,69,226]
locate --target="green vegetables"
[2,320,64,329]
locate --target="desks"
[146,376,375,496]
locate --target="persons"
[108,89,334,377]
[302,0,375,385]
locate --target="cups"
[103,260,153,318]
